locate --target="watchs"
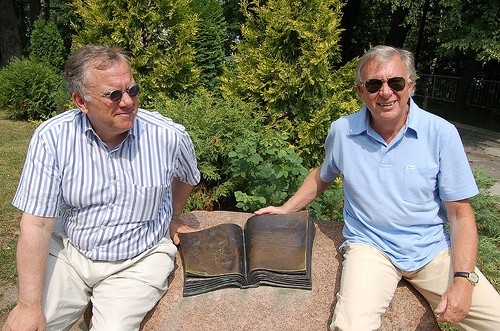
[453,272,479,284]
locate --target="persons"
[1,44,200,331]
[255,46,500,331]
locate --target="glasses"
[361,76,411,94]
[89,83,141,102]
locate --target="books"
[179,209,314,297]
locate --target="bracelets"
[172,211,183,220]
